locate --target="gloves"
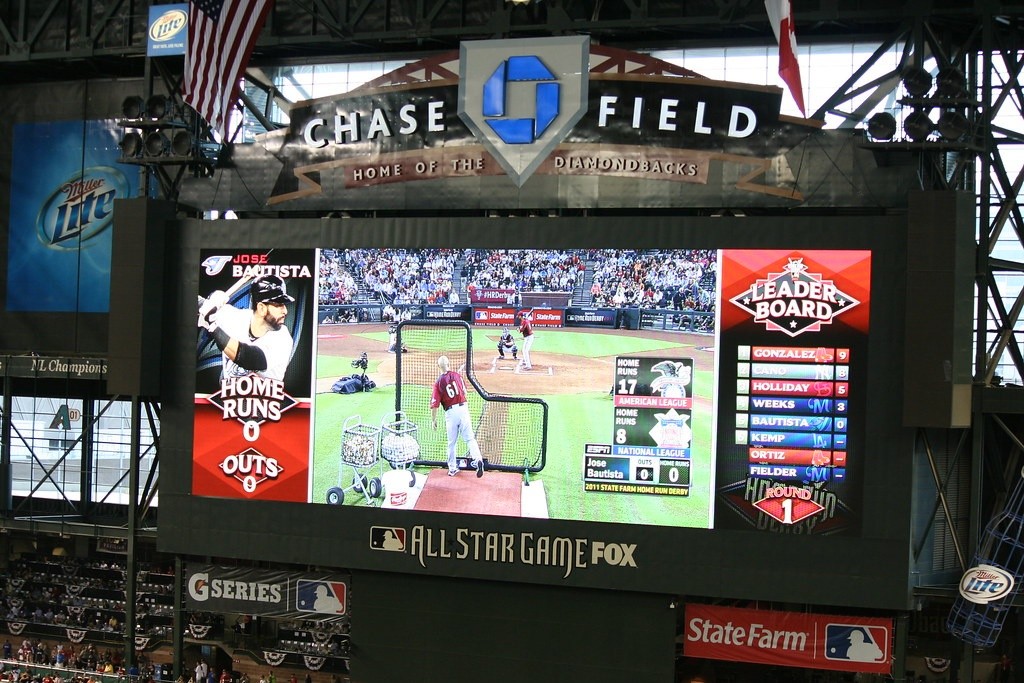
[196,295,227,337]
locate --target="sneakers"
[475,459,485,477]
[449,467,459,476]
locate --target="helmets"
[503,327,510,332]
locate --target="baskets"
[380,421,421,465]
[341,423,382,468]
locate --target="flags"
[681,602,893,674]
[183,0,274,144]
[762,0,807,120]
[186,561,350,627]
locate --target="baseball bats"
[516,307,535,331]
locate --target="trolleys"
[326,414,384,506]
[368,411,421,499]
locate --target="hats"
[515,311,523,317]
[250,273,296,307]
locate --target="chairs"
[317,248,719,336]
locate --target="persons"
[0,549,349,683]
[498,327,517,360]
[199,276,294,387]
[319,249,716,330]
[429,355,484,479]
[515,311,533,369]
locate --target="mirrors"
[308,250,723,531]
